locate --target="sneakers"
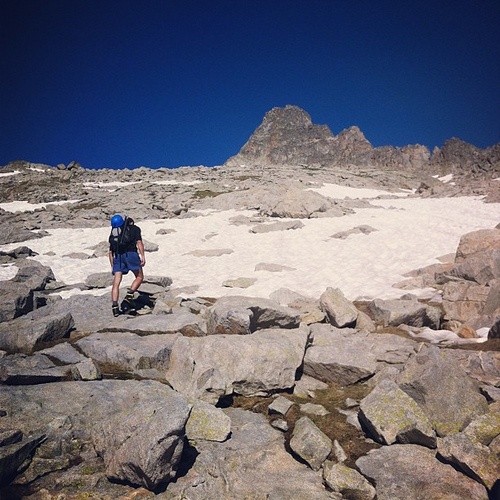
[121,299,136,313]
[112,305,121,317]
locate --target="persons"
[108,217,145,317]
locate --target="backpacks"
[109,216,136,253]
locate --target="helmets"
[111,215,123,227]
